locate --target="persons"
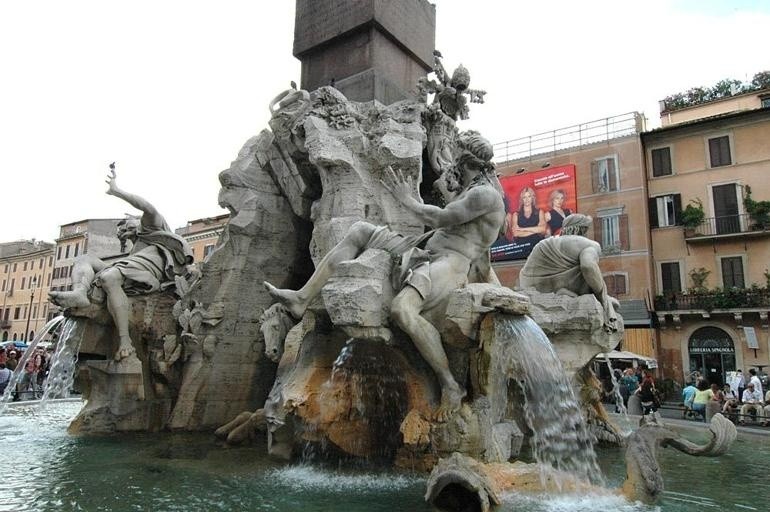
[682,378,699,410]
[749,368,763,394]
[0,342,53,401]
[719,383,740,418]
[518,214,622,316]
[610,364,658,417]
[737,382,769,427]
[708,382,721,402]
[43,167,195,365]
[690,379,715,419]
[507,187,548,246]
[542,189,573,236]
[762,389,770,427]
[262,131,508,425]
[735,369,747,403]
[494,195,510,245]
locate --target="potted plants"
[677,193,704,236]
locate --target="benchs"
[659,404,740,424]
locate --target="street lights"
[22,272,39,347]
